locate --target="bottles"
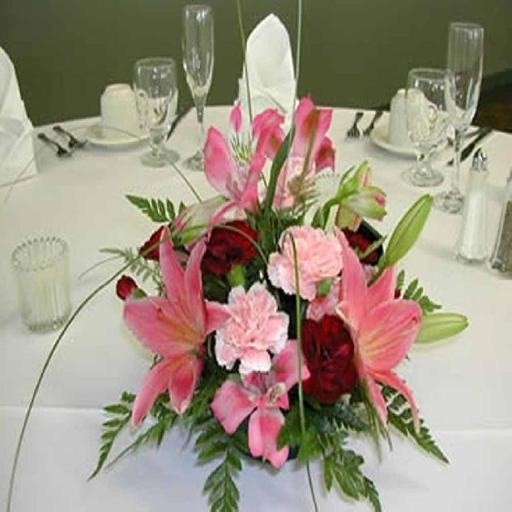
[486,168,512,276]
[456,147,490,260]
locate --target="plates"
[84,118,149,151]
[370,123,454,154]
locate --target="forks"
[346,111,364,139]
[51,125,88,152]
[37,133,75,159]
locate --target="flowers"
[8,1,468,511]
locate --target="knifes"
[363,108,384,137]
[446,123,494,167]
[165,102,194,144]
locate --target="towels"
[236,14,297,118]
[0,48,38,188]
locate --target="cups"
[100,81,149,141]
[388,90,439,148]
[131,55,181,170]
[10,234,73,336]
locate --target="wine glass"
[400,66,458,190]
[432,21,486,217]
[180,3,216,172]
